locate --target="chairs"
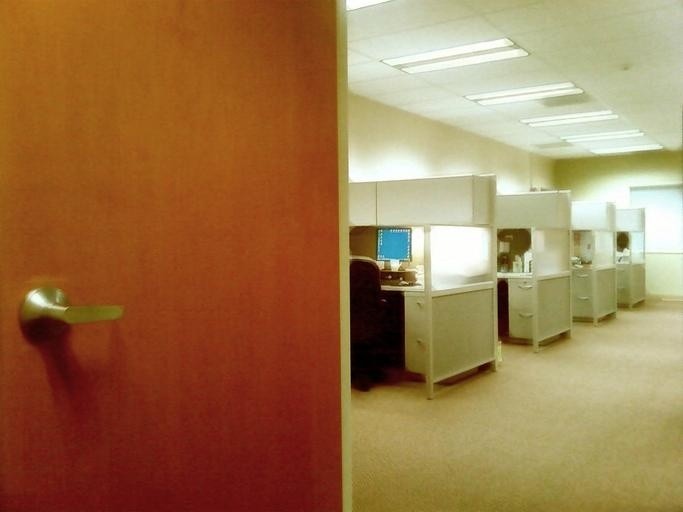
[350,256,388,391]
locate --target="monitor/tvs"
[376,227,412,270]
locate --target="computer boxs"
[379,267,418,287]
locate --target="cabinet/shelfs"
[497,190,572,353]
[617,208,645,308]
[348,174,497,400]
[571,200,617,325]
